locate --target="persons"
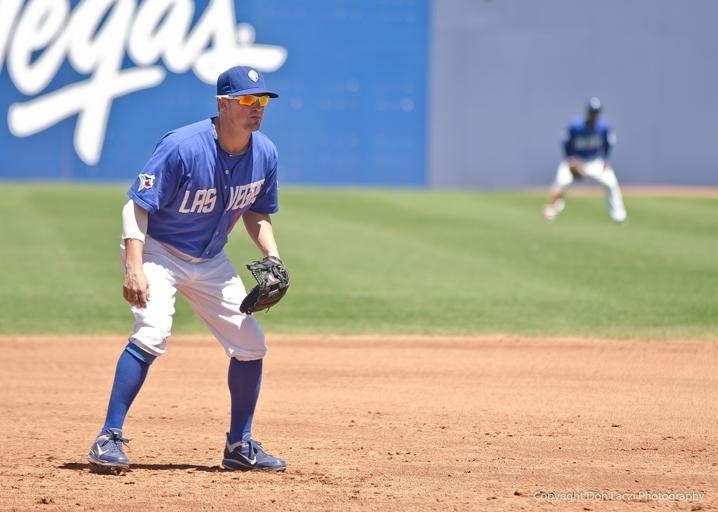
[544,95,629,223]
[87,66,289,471]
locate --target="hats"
[216,65,279,98]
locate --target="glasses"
[219,95,269,106]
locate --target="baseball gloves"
[239,259,290,314]
[570,163,586,181]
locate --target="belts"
[159,241,208,264]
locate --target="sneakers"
[222,432,286,471]
[87,428,131,475]
